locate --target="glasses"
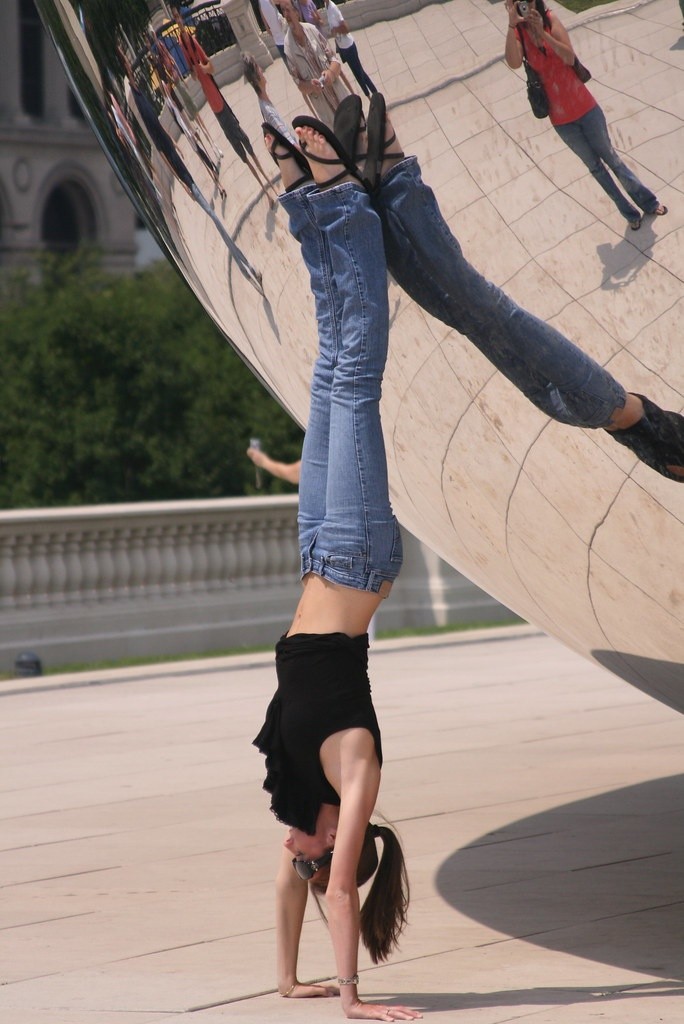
[291,854,337,880]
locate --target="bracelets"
[338,975,359,984]
[509,24,515,29]
[280,985,294,996]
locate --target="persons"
[240,51,301,151]
[168,0,279,211]
[324,0,378,100]
[252,116,422,1022]
[116,46,264,296]
[334,93,684,483]
[275,0,351,131]
[504,0,668,230]
[144,24,228,200]
[298,0,324,30]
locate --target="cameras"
[318,76,325,88]
[518,1,530,16]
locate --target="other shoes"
[214,147,227,199]
[249,267,262,282]
[247,277,263,296]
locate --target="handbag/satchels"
[545,15,592,83]
[516,24,551,119]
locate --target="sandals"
[260,119,314,193]
[655,205,668,215]
[293,115,374,196]
[332,96,368,173]
[359,92,405,190]
[631,219,640,231]
